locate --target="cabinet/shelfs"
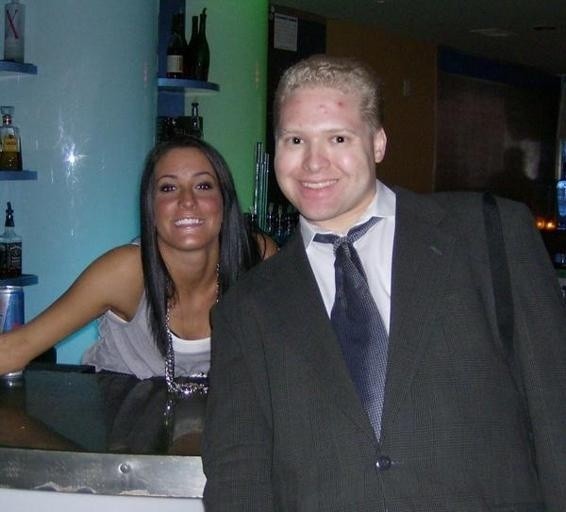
[326,20,439,200]
[436,75,509,194]
[0,59,40,288]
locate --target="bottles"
[3,1,26,64]
[249,211,301,246]
[154,101,205,146]
[0,201,24,279]
[166,10,211,81]
[0,104,24,172]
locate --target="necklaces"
[163,264,223,421]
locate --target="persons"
[201,54,566,512]
[0,135,282,381]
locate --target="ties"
[314,219,393,446]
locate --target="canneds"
[0,286,26,380]
[5,381,27,413]
